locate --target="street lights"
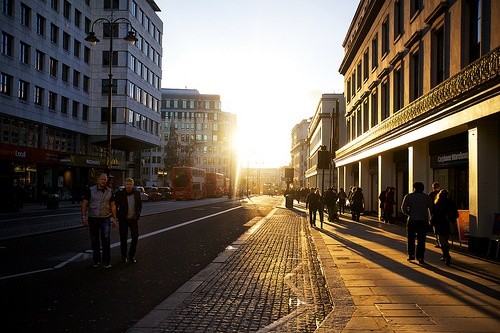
[83,10,139,192]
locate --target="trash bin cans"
[285,193,293,209]
[46,191,60,209]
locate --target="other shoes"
[92,261,100,267]
[103,263,112,268]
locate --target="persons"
[427,182,444,248]
[250,185,366,228]
[80,172,117,269]
[1,175,94,207]
[400,181,435,264]
[379,186,392,221]
[384,186,397,224]
[429,189,461,264]
[114,177,142,264]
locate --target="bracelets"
[81,216,86,219]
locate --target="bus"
[237,179,286,199]
[204,172,224,198]
[223,177,230,196]
[171,166,207,202]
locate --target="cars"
[158,187,173,201]
[144,186,162,202]
[117,185,148,202]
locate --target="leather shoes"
[129,256,137,263]
[121,258,128,265]
